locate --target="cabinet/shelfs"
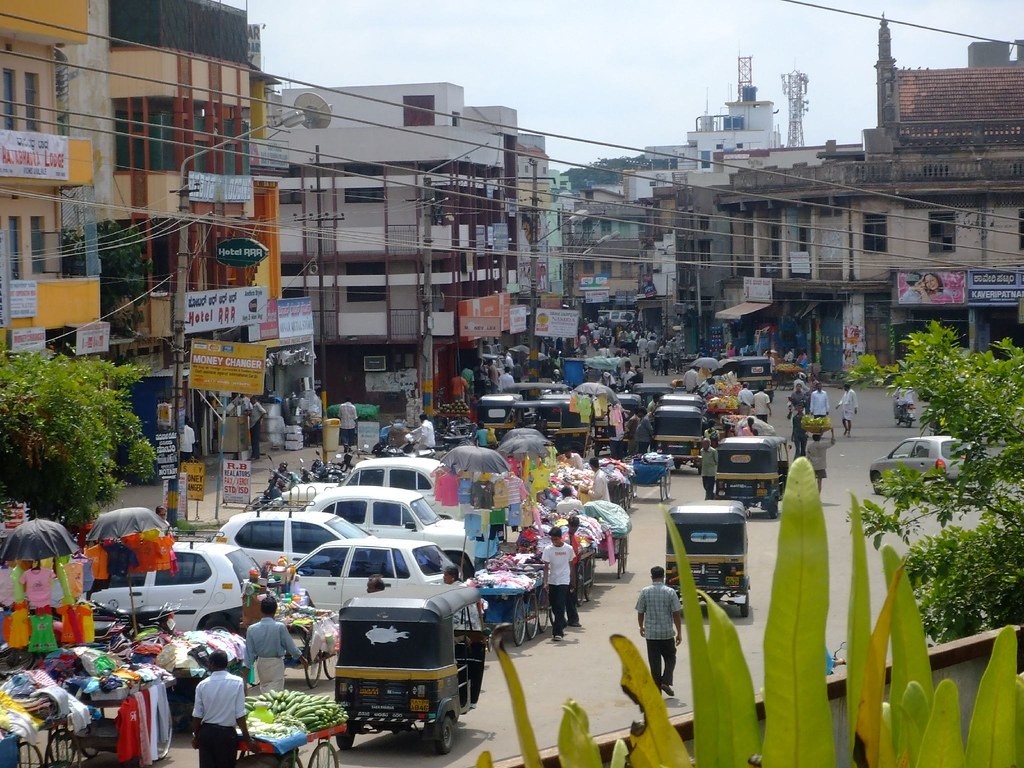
[218,416,249,452]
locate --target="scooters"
[891,396,917,428]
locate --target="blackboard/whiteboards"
[357,421,380,455]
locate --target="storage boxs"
[285,425,302,434]
[287,433,304,442]
[284,441,303,451]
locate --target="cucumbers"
[245,689,349,732]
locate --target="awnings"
[714,302,772,321]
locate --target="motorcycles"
[241,331,715,511]
[75,594,182,646]
[714,434,794,520]
[331,582,492,756]
[0,598,135,683]
[661,498,751,618]
[709,355,774,404]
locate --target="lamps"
[345,336,359,341]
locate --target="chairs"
[918,449,930,457]
[351,551,372,578]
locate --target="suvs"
[74,529,263,636]
[210,507,378,573]
[338,456,474,522]
[304,483,476,582]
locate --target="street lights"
[567,230,622,309]
[528,207,591,400]
[165,107,308,528]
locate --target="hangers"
[435,465,447,471]
[442,463,458,476]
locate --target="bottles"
[280,593,308,606]
[325,633,335,652]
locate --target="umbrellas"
[690,356,722,370]
[86,507,173,638]
[439,426,553,475]
[575,381,621,456]
[1,519,80,561]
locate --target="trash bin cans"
[325,418,341,452]
[0,735,20,768]
[681,359,697,376]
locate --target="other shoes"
[554,636,562,640]
[568,622,582,627]
[844,429,848,435]
[848,432,851,438]
[344,444,348,453]
[247,457,256,460]
[661,683,674,696]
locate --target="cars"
[869,434,985,495]
[290,537,462,613]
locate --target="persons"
[179,415,195,462]
[408,414,436,451]
[365,574,387,593]
[443,567,484,712]
[449,318,915,515]
[541,516,580,642]
[540,265,547,290]
[474,523,500,572]
[248,396,267,460]
[899,272,954,303]
[634,565,682,697]
[154,505,167,520]
[190,650,261,768]
[824,642,844,675]
[338,396,358,453]
[241,596,309,697]
[581,457,611,502]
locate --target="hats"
[708,419,716,425]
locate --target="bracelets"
[192,732,196,737]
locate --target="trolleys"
[471,547,597,648]
[191,718,349,768]
[771,366,827,391]
[576,501,631,580]
[251,608,340,690]
[68,674,177,763]
